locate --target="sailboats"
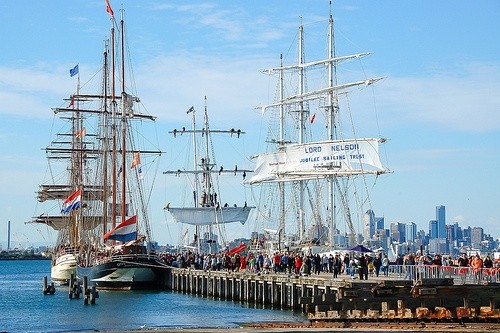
[23,4,170,292]
[243,0,395,264]
[163,97,255,257]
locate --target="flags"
[61,190,81,213]
[66,98,74,108]
[106,0,113,15]
[131,152,140,168]
[103,216,137,242]
[118,166,122,176]
[310,114,315,123]
[76,128,86,138]
[70,65,79,77]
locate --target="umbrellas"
[344,245,373,253]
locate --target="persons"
[250,235,266,246]
[395,253,500,282]
[194,234,197,242]
[159,250,390,281]
